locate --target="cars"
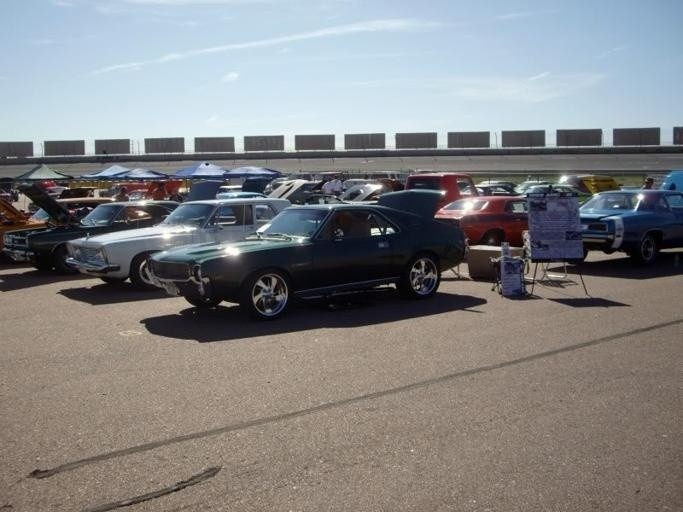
[567,170,683,265]
[523,184,593,205]
[216,179,308,199]
[0,197,116,251]
[64,198,291,290]
[128,189,148,200]
[462,184,519,196]
[145,189,466,321]
[2,186,180,275]
[434,196,529,246]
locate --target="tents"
[167,163,226,180]
[106,169,168,180]
[81,166,131,178]
[14,163,73,179]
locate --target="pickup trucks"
[404,172,480,210]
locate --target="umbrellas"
[223,167,281,179]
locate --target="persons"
[170,190,182,202]
[332,176,341,197]
[152,183,168,200]
[116,187,129,202]
[322,178,333,195]
[641,178,654,189]
